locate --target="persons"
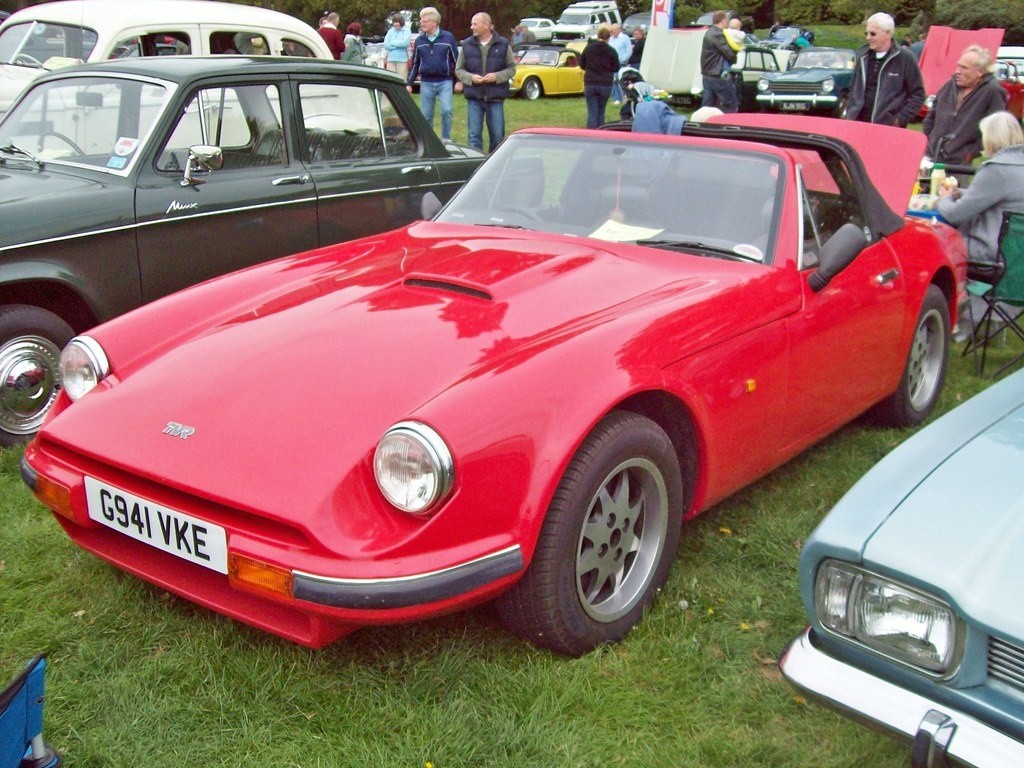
[719,18,745,81]
[700,10,740,115]
[769,22,779,39]
[937,110,1024,345]
[406,7,463,141]
[793,36,810,49]
[580,23,621,128]
[847,12,927,129]
[317,12,647,106]
[917,45,1008,195]
[455,12,517,154]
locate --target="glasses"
[420,20,430,23]
[863,30,877,37]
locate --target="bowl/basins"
[909,194,941,212]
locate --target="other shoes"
[614,100,620,104]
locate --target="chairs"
[961,209,1024,380]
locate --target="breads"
[944,176,958,190]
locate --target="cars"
[505,2,1024,122]
[775,365,1024,768]
[18,110,971,658]
[0,52,489,450]
[1,0,384,159]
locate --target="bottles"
[930,162,946,196]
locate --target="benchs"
[254,130,411,160]
[156,147,281,173]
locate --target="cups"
[918,178,931,194]
